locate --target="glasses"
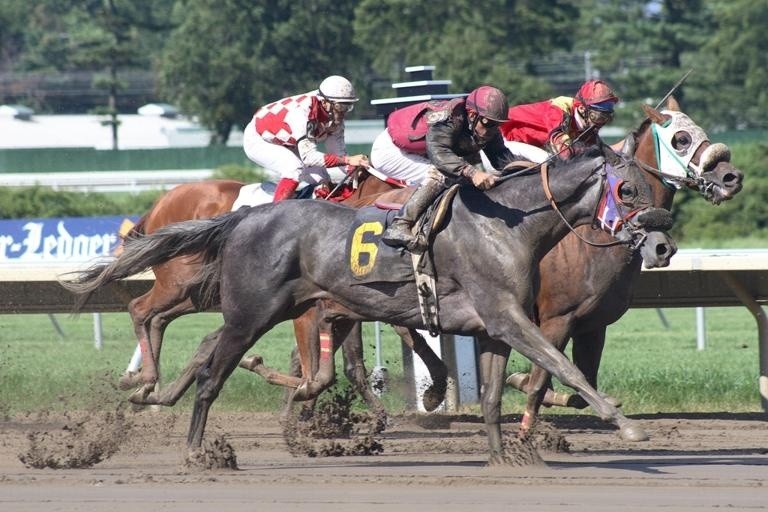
[474,88,497,129]
[579,90,611,126]
[322,92,354,112]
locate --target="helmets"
[573,81,618,106]
[319,75,359,104]
[465,86,511,123]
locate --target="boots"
[382,186,436,252]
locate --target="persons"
[241,75,370,205]
[497,81,619,165]
[368,84,538,251]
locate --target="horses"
[56,94,745,469]
